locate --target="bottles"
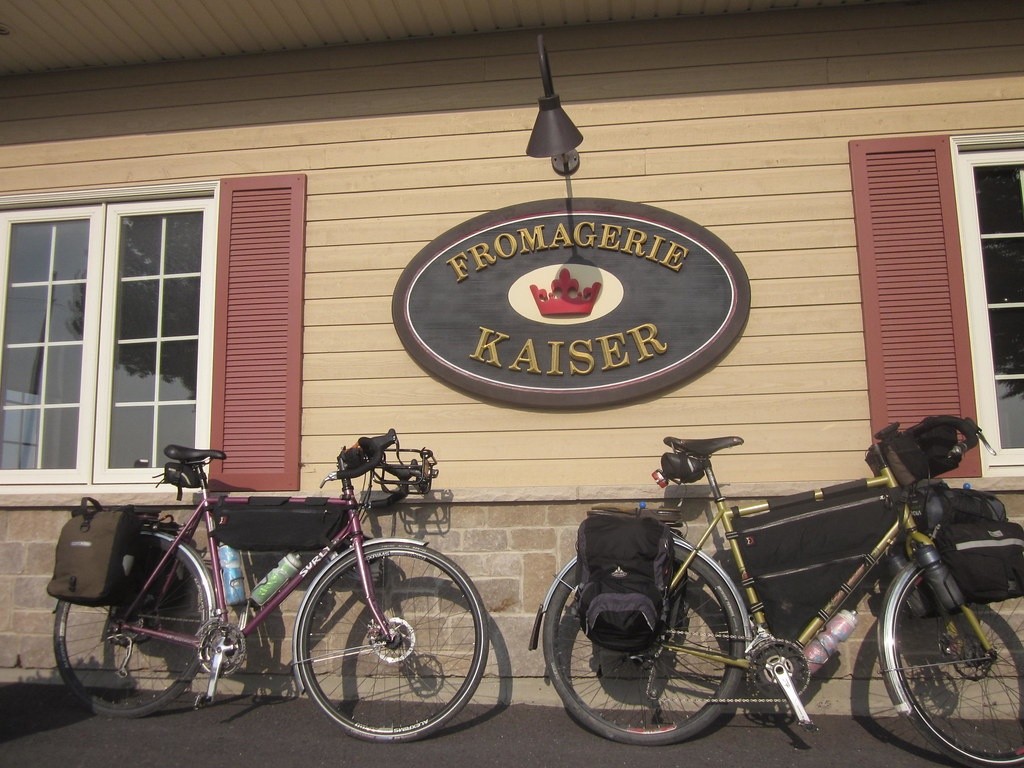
[247,553,303,609]
[216,541,248,605]
[802,609,859,680]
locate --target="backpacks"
[914,484,1024,604]
[573,509,675,651]
[47,496,129,607]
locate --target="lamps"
[525,34,583,175]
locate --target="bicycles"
[53,428,490,744]
[527,414,1024,768]
